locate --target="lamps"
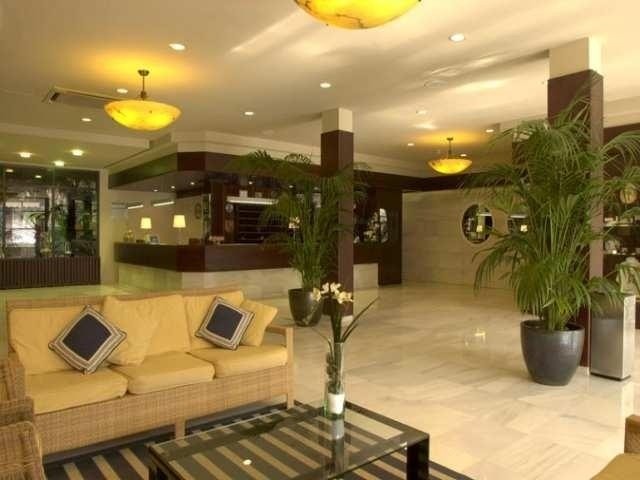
[102,69,182,132]
[172,214,187,244]
[425,138,473,175]
[139,217,151,230]
[288,216,300,240]
[292,0,423,32]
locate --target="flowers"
[309,280,381,393]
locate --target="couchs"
[0,288,296,458]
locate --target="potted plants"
[223,147,372,328]
[458,68,640,388]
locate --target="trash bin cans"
[590,291,636,379]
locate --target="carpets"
[44,399,478,480]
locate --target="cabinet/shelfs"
[112,240,379,302]
[235,205,288,244]
[511,122,640,332]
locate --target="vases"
[324,380,346,420]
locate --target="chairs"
[588,413,640,480]
[1,419,49,479]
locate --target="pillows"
[239,299,278,348]
[101,295,160,368]
[48,303,128,376]
[194,295,254,352]
